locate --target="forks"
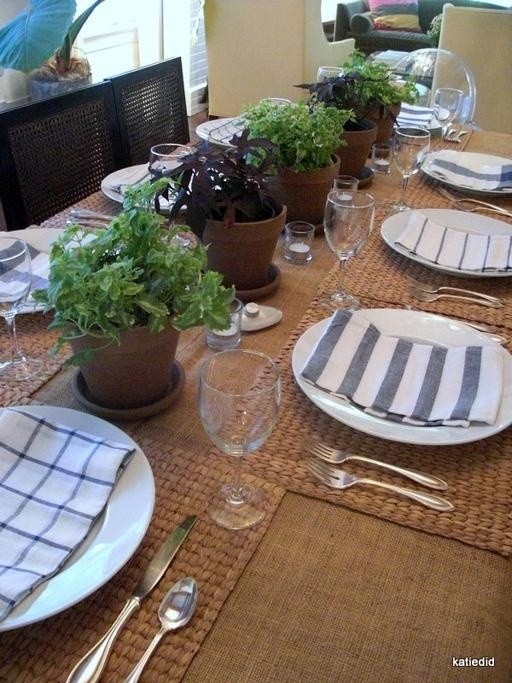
[300,434,456,491]
[401,271,507,308]
[303,457,460,515]
[437,185,511,216]
[444,126,469,145]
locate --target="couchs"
[336,1,512,50]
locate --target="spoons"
[124,568,197,681]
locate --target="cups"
[205,296,244,350]
[282,220,316,264]
[332,175,360,190]
[315,64,343,83]
[262,96,292,107]
[372,142,391,173]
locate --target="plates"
[0,402,159,635]
[290,308,511,446]
[100,160,267,214]
[196,116,248,149]
[389,79,430,97]
[381,207,512,278]
[420,149,512,196]
[0,229,102,314]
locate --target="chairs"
[104,56,190,165]
[0,80,126,231]
[430,3,510,132]
[203,1,356,117]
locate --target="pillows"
[368,0,421,32]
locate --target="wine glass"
[149,143,193,248]
[384,124,432,210]
[0,235,42,382]
[432,87,465,154]
[195,349,283,528]
[316,189,377,311]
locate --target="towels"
[301,307,504,429]
[393,103,433,130]
[0,237,64,306]
[393,210,512,272]
[209,120,246,144]
[0,407,137,621]
[430,149,512,189]
[112,167,155,197]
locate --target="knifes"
[63,513,197,683]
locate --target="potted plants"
[32,50,419,422]
[233,98,357,241]
[340,49,419,158]
[292,73,400,191]
[148,141,287,304]
[32,181,236,424]
